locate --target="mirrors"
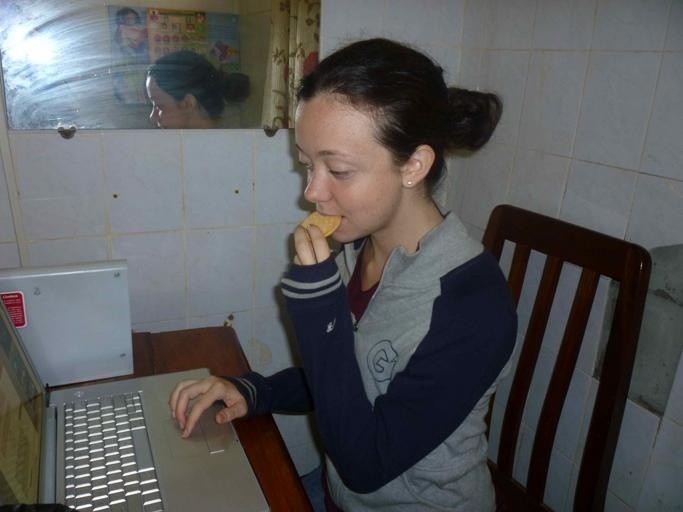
[0,0,322,130]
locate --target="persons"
[167,35,519,512]
[145,51,250,128]
[115,7,147,58]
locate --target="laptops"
[0,296,270,512]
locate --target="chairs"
[480,203,653,509]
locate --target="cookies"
[299,211,342,241]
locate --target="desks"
[49,327,311,510]
